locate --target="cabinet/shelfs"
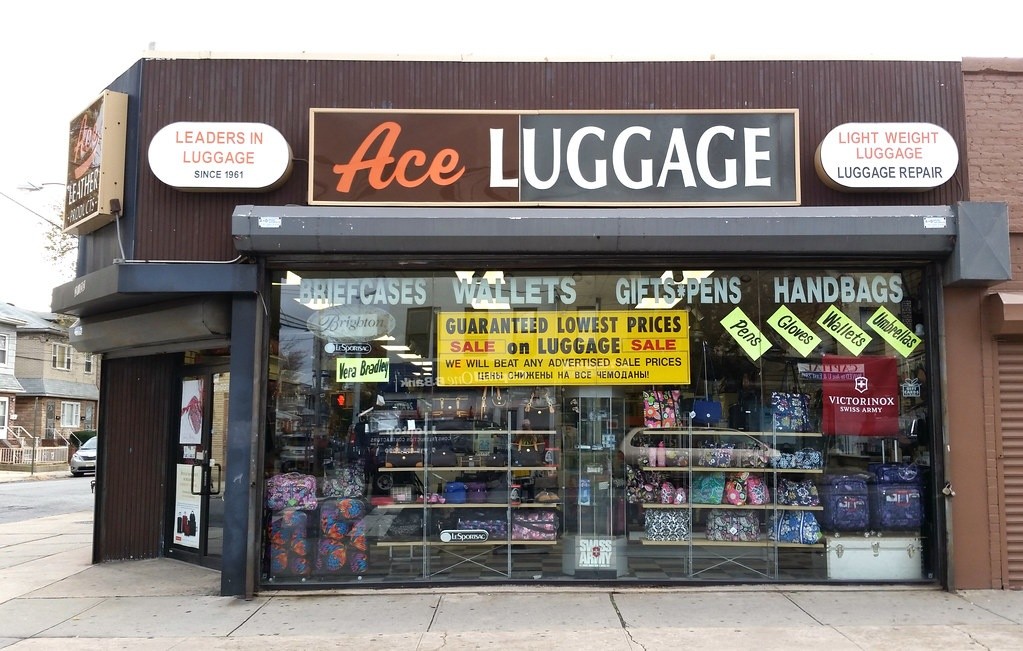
[373,426,557,583]
[637,429,826,572]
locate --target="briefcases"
[824,531,924,583]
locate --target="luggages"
[820,471,872,538]
[262,498,370,581]
[871,460,926,538]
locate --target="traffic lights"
[331,392,345,407]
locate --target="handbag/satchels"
[726,392,814,429]
[704,507,762,542]
[462,479,487,503]
[691,397,723,424]
[765,509,825,544]
[321,455,368,501]
[510,508,561,542]
[643,507,690,542]
[266,471,317,512]
[484,452,507,467]
[441,481,470,505]
[477,470,508,505]
[518,446,546,468]
[383,443,424,469]
[615,434,826,531]
[455,511,508,538]
[642,386,683,431]
[430,446,460,468]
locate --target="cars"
[364,414,543,496]
[69,435,98,478]
[279,433,345,475]
[615,425,781,490]
